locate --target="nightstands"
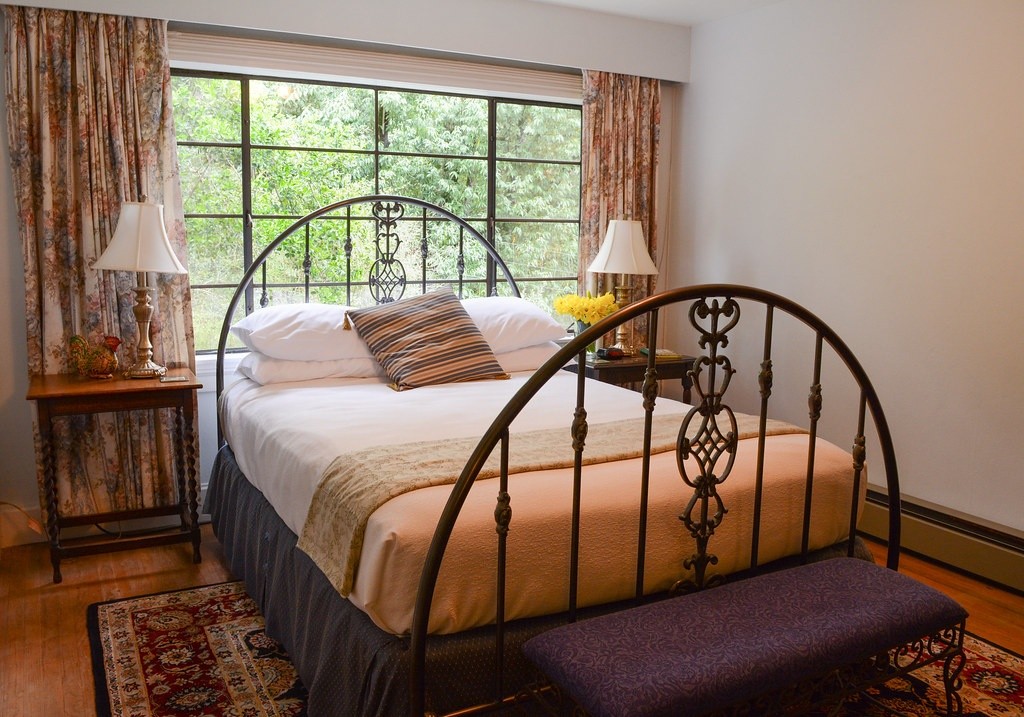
[26,367,203,584]
[570,351,698,405]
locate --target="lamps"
[588,219,660,357]
[90,194,188,380]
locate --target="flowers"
[552,290,619,326]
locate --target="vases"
[575,319,590,334]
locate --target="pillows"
[457,296,569,355]
[346,283,510,392]
[236,350,388,384]
[228,303,377,361]
[494,341,577,372]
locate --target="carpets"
[87,578,1024,716]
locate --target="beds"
[204,195,902,717]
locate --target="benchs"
[521,560,967,717]
